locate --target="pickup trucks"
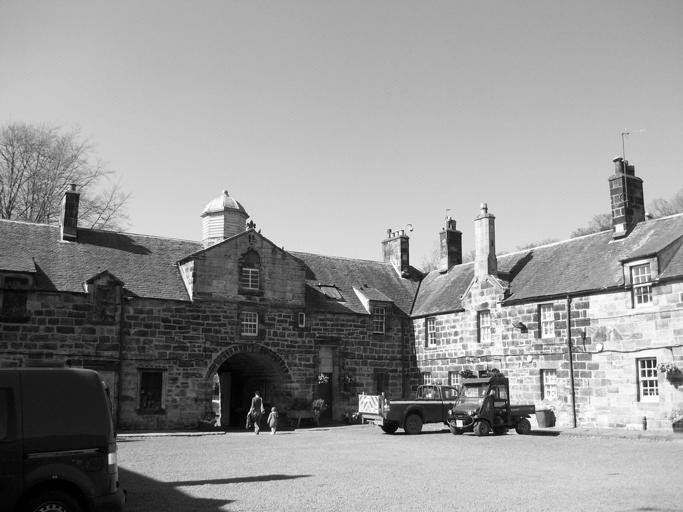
[356,382,461,434]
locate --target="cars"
[445,375,536,437]
[1,365,128,512]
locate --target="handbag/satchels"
[246,413,254,430]
[260,398,265,414]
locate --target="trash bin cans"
[535,409,552,428]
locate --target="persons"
[246,390,265,435]
[266,406,279,434]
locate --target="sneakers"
[255,427,260,435]
